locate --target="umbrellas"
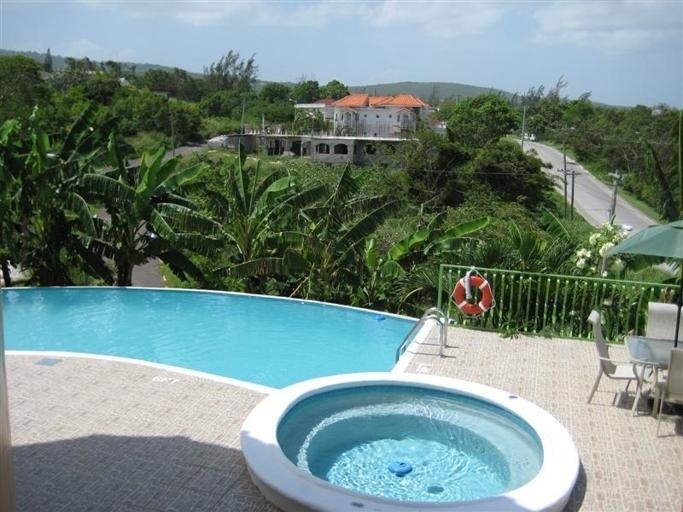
[601,217,683,348]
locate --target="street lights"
[289,98,297,121]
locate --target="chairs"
[586,301,683,438]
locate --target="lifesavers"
[455,277,491,315]
